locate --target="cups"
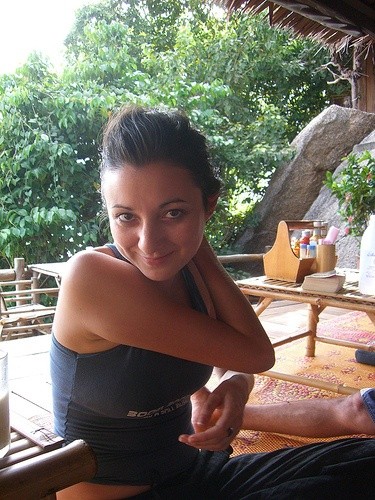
[316,244,337,272]
[0,349,10,460]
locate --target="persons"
[50,103,375,500]
[190,384,375,438]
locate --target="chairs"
[0,280,59,343]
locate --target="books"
[302,273,346,292]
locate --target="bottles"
[359,215,375,295]
[299,222,328,257]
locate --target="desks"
[27,263,68,305]
[232,266,375,359]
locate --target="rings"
[226,426,234,437]
